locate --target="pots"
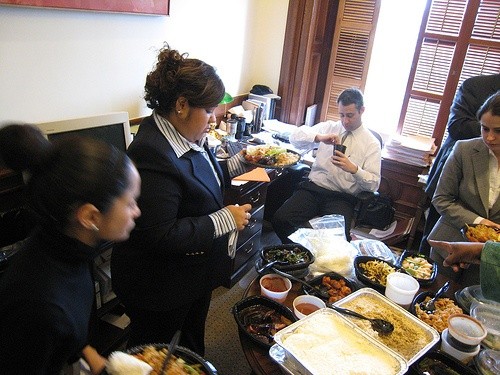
[81,342,220,375]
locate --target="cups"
[226,114,245,140]
[332,143,347,165]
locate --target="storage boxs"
[94,247,117,309]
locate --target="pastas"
[358,258,396,285]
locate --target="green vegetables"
[405,254,435,279]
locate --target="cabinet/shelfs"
[218,142,314,288]
[90,241,129,357]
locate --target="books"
[243,92,278,132]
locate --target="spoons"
[325,302,394,334]
[255,258,289,273]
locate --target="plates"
[240,148,301,169]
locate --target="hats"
[250,84,281,101]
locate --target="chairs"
[287,129,383,228]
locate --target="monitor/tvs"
[37,111,132,152]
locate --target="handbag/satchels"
[355,191,396,230]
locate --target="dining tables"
[239,246,480,375]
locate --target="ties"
[341,130,352,145]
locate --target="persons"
[0,124,141,374]
[427,89,500,279]
[271,88,382,245]
[124,48,259,358]
[416,73,500,257]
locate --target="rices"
[415,298,463,332]
[275,152,299,165]
[343,297,431,359]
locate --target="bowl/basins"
[260,274,292,303]
[259,243,316,276]
[461,223,500,243]
[273,287,440,375]
[292,295,326,320]
[302,270,357,308]
[354,255,411,295]
[398,248,438,287]
[384,273,420,306]
[412,284,500,375]
[232,293,299,350]
